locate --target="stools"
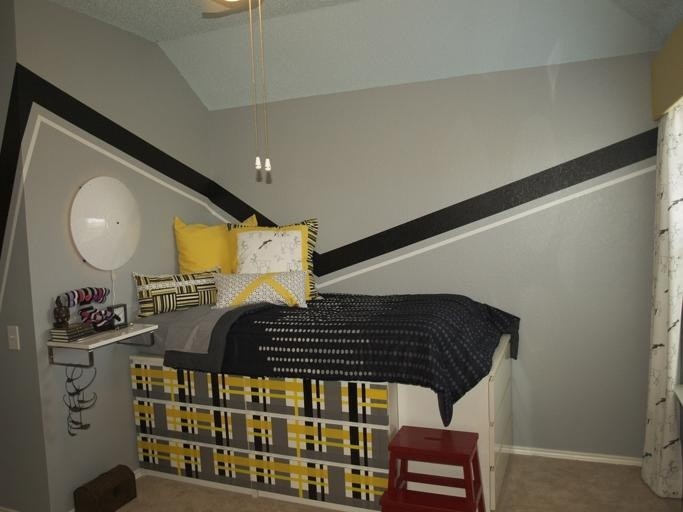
[378,425,488,512]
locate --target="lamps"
[69,175,144,305]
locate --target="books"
[47,324,93,343]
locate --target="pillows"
[131,213,324,318]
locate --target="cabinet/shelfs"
[398,333,513,512]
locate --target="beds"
[122,295,514,512]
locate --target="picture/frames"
[108,304,127,330]
